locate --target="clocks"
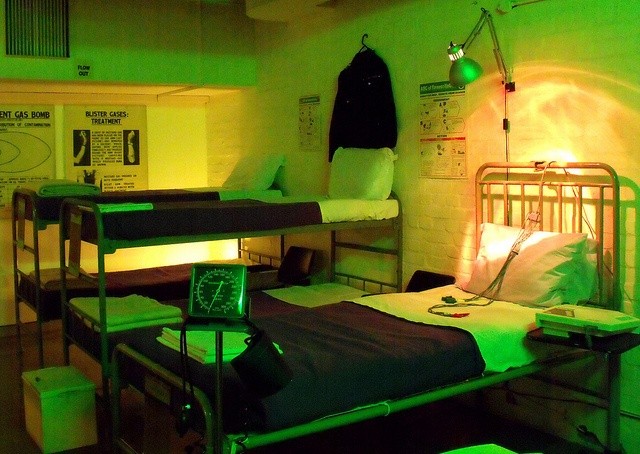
[186,259,246,321]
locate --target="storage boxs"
[19,364,97,454]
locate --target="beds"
[10,183,285,355]
[60,191,403,445]
[108,160,640,454]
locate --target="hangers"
[356,34,369,54]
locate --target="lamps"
[446,7,515,95]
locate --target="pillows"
[454,223,589,308]
[325,145,399,200]
[579,238,596,302]
[222,155,288,191]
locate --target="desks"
[527,326,640,453]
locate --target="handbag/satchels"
[232,329,294,402]
